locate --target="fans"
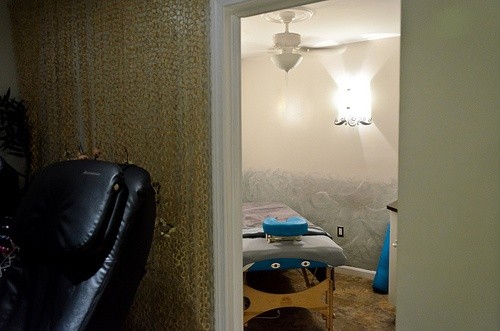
[241,7,401,74]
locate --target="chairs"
[0,160,156,331]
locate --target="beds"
[242,202,347,331]
